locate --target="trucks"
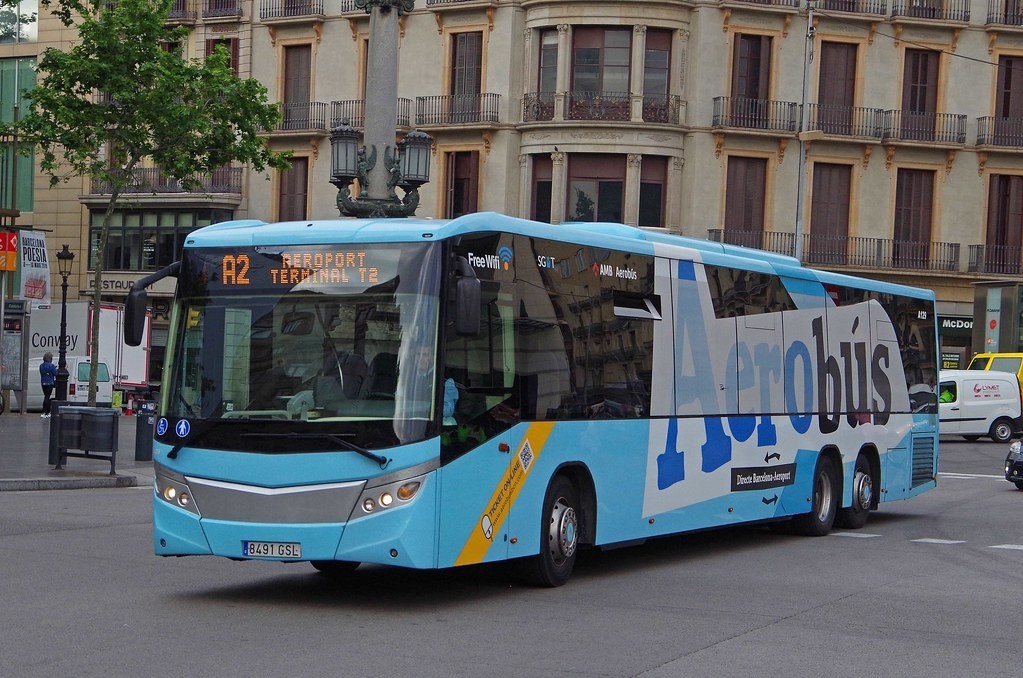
[28,300,151,407]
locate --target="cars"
[1003,437,1023,491]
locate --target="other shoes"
[40,413,52,419]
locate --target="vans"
[0,356,113,412]
[938,370,1023,444]
[966,353,1023,408]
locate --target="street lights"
[55,244,75,400]
[329,123,433,220]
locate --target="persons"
[939,386,954,402]
[39,352,56,419]
[409,341,459,417]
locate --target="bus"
[124,211,940,588]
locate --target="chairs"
[365,351,396,402]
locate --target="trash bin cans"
[78,408,119,452]
[57,406,95,450]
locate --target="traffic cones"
[126,393,133,416]
